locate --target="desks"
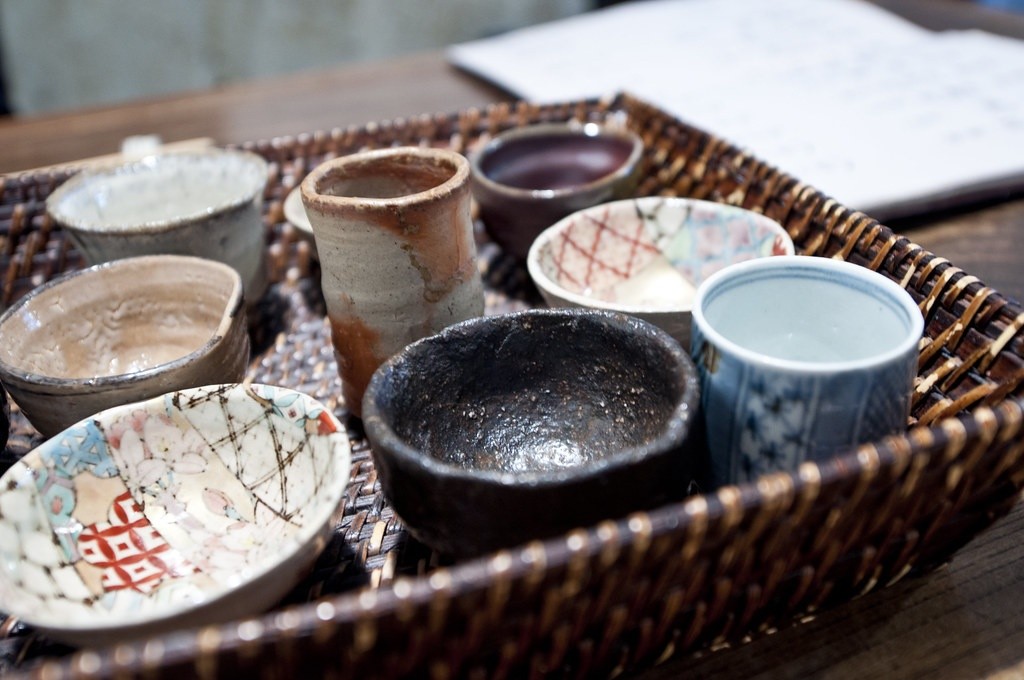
[1,0,1022,680]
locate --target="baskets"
[0,94,1024,680]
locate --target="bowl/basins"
[527,196,795,357]
[362,308,703,559]
[0,383,352,647]
[468,123,646,264]
[0,255,251,439]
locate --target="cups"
[301,145,485,418]
[690,255,924,494]
[45,146,269,307]
